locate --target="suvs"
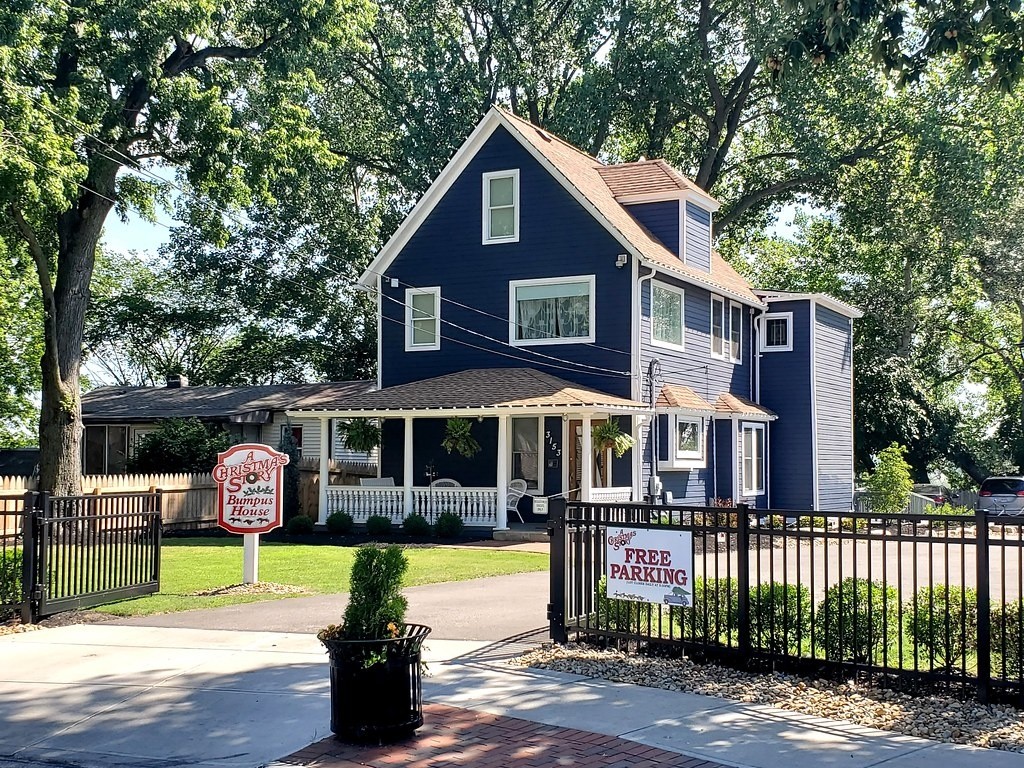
[973,476,1024,516]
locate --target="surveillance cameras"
[615,261,623,269]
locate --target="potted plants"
[590,416,637,460]
[313,532,435,748]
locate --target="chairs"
[493,478,528,524]
[359,477,397,486]
[427,477,461,487]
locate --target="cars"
[912,483,960,509]
